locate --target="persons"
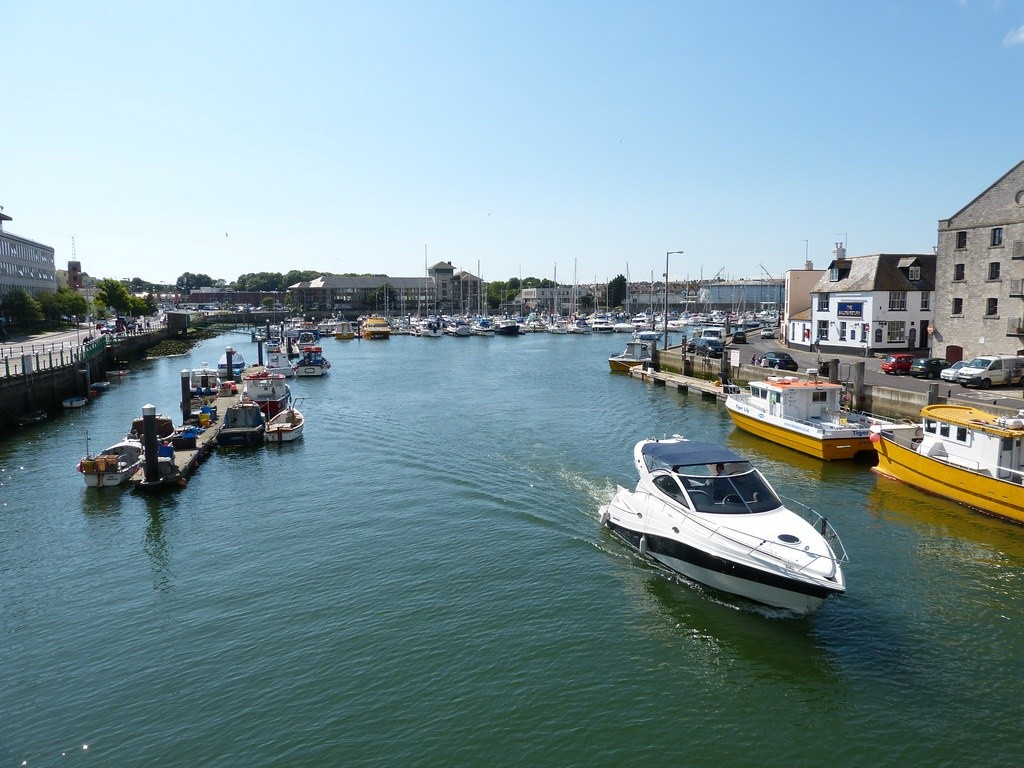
[660,467,691,490]
[815,336,820,353]
[713,464,728,502]
[749,353,769,367]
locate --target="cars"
[731,331,748,344]
[756,352,798,372]
[761,328,774,339]
[686,327,726,358]
[162,305,240,311]
[909,358,952,379]
[940,361,970,382]
[96,316,127,334]
[881,354,915,375]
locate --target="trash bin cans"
[818,361,829,376]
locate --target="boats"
[608,338,652,371]
[189,316,391,395]
[868,404,1024,526]
[80,413,175,487]
[725,369,898,460]
[14,371,127,424]
[598,433,851,617]
[216,369,304,445]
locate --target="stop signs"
[866,325,869,330]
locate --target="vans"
[956,355,1024,389]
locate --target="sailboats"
[411,244,777,343]
